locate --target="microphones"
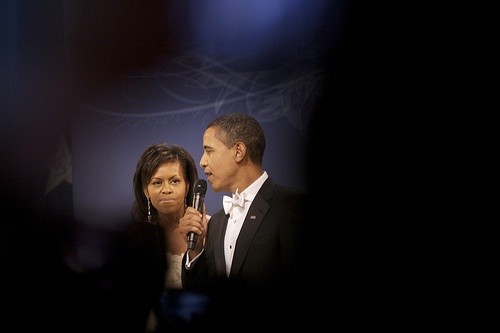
[187,178,208,251]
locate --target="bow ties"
[222,188,244,215]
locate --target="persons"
[181,112,306,333]
[130,144,212,333]
